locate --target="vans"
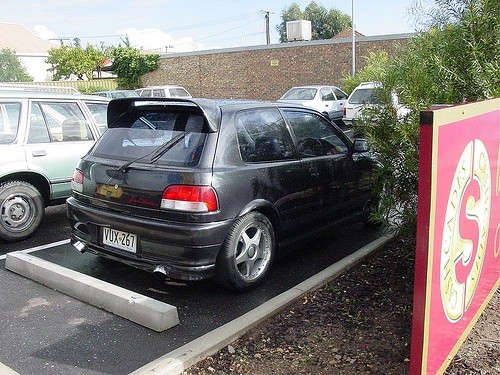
[92,85,194,118]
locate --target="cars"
[276,86,350,120]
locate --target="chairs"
[255,136,281,160]
[62,117,87,141]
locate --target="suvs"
[66,97,395,292]
[342,81,411,127]
[0,84,172,242]
